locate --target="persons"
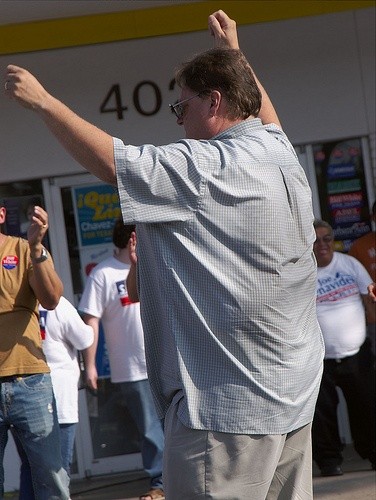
[21,201,35,233]
[78,214,164,500]
[350,199,376,358]
[312,219,376,476]
[0,200,71,500]
[9,294,95,500]
[4,9,325,500]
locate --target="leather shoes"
[320,465,343,477]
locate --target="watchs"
[30,247,47,263]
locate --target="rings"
[5,81,8,89]
[43,224,47,228]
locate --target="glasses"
[168,94,199,119]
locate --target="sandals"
[138,488,165,500]
